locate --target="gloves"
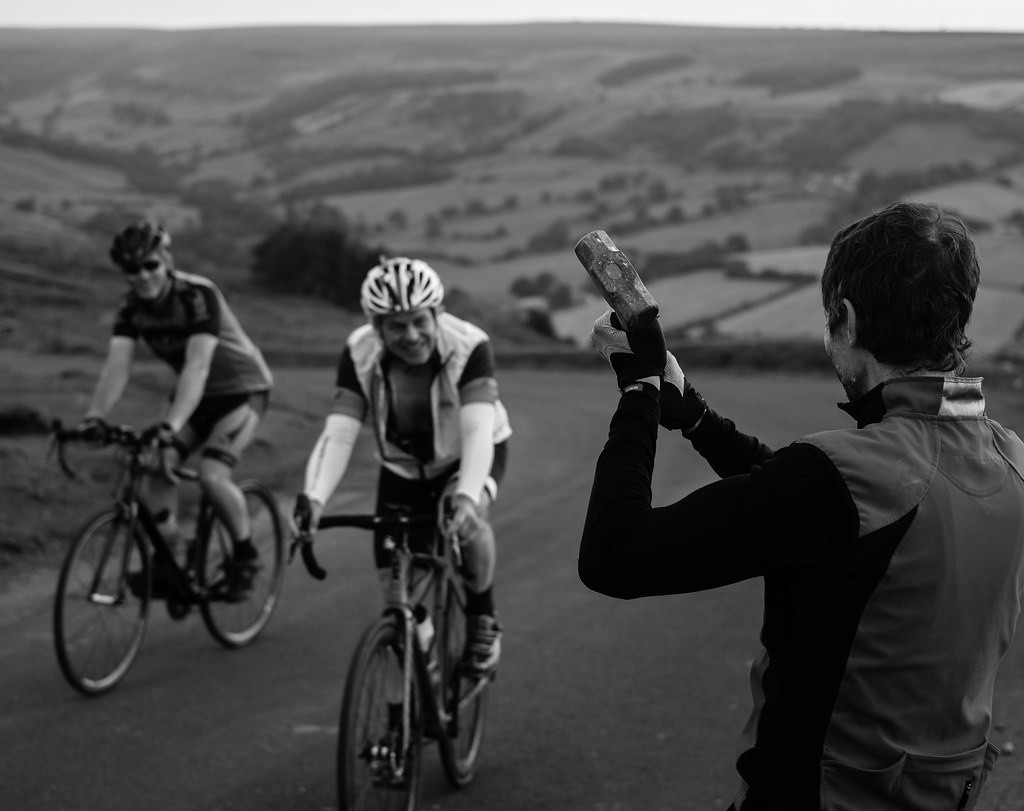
[591,310,708,431]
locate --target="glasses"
[124,259,162,274]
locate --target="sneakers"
[463,616,502,669]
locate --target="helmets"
[111,223,168,270]
[360,256,444,322]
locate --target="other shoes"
[230,552,262,593]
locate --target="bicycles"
[283,509,498,810]
[51,424,286,699]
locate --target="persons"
[83,221,274,602]
[290,257,513,786]
[576,201,1024,808]
